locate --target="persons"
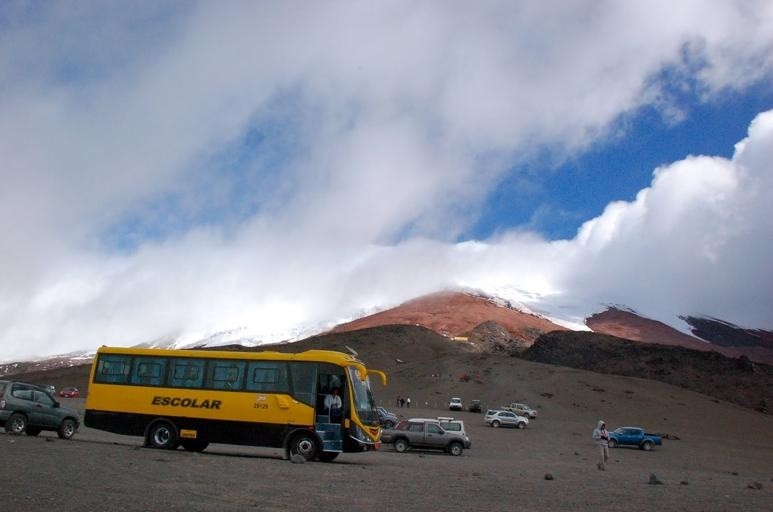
[322,386,342,417]
[405,395,410,408]
[591,419,610,471]
[394,395,401,409]
[399,398,405,407]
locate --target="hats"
[60,386,81,398]
[486,410,529,430]
[0,377,81,441]
[380,421,471,456]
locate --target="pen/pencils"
[408,416,466,437]
[449,397,463,410]
[376,409,399,428]
[39,385,57,396]
[484,408,499,423]
[377,406,395,416]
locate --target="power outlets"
[500,402,537,419]
[607,426,662,451]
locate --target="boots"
[468,399,483,413]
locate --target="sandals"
[83,345,389,463]
